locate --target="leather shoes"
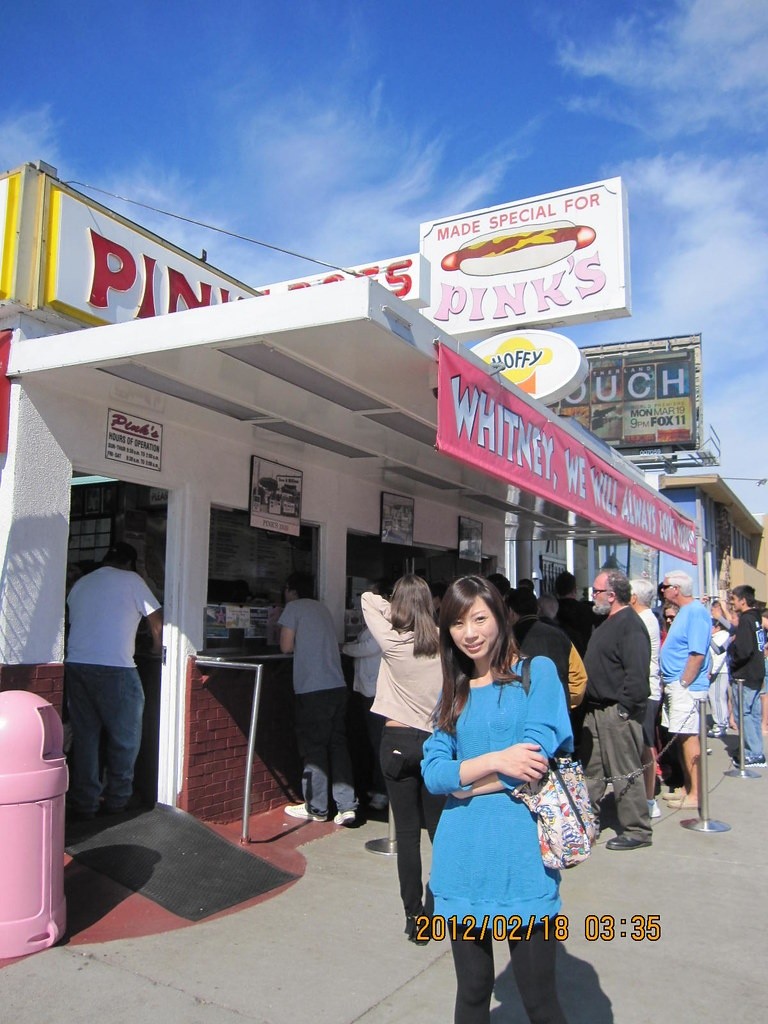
[606,836,653,850]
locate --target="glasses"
[592,587,607,594]
[662,585,673,589]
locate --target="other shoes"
[663,787,701,810]
[648,800,660,818]
[367,791,388,810]
[732,755,767,768]
[405,912,430,946]
[709,728,727,737]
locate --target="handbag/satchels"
[510,655,597,870]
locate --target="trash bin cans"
[0,690,68,959]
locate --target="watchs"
[679,678,688,688]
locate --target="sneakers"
[334,810,355,825]
[285,802,328,822]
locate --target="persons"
[421,574,575,1024]
[65,541,163,818]
[362,576,441,945]
[278,571,359,826]
[339,569,768,851]
